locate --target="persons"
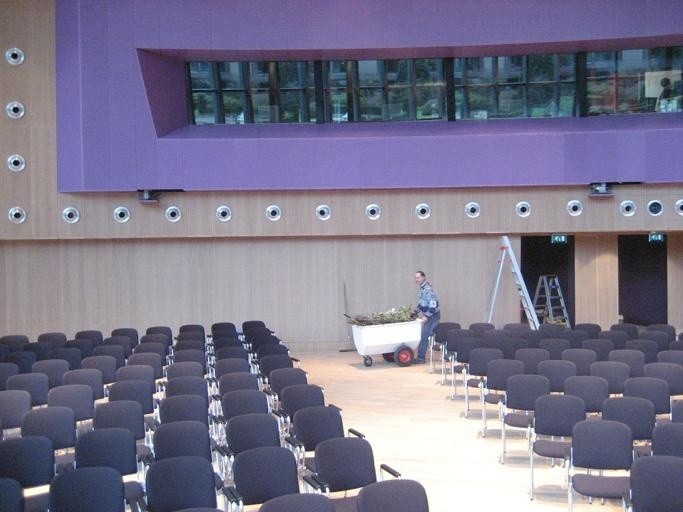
[660,77,674,99]
[410,271,441,363]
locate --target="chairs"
[539,338,570,361]
[63,369,109,399]
[212,358,257,380]
[521,329,552,348]
[216,373,274,408]
[158,376,218,414]
[222,414,301,477]
[531,394,586,498]
[649,421,683,456]
[242,320,265,343]
[623,455,683,512]
[539,360,576,395]
[48,348,80,369]
[105,335,134,357]
[601,396,655,503]
[229,446,323,511]
[0,343,9,355]
[212,338,248,349]
[209,322,237,331]
[609,349,645,376]
[49,466,137,511]
[430,323,461,374]
[600,332,628,347]
[562,349,595,376]
[483,329,511,348]
[163,362,213,392]
[1,436,65,488]
[258,493,334,511]
[569,418,633,505]
[466,348,504,418]
[564,376,611,421]
[442,331,478,386]
[2,335,30,349]
[668,342,683,349]
[251,334,275,357]
[147,326,176,345]
[0,389,38,430]
[501,375,550,464]
[171,340,212,361]
[482,359,523,438]
[116,365,162,393]
[94,343,130,367]
[7,349,35,373]
[626,340,658,363]
[214,328,240,339]
[670,396,682,421]
[472,323,494,335]
[134,343,172,366]
[293,406,366,473]
[574,323,601,338]
[178,325,208,341]
[156,394,218,437]
[23,340,52,361]
[219,390,285,436]
[169,349,211,370]
[73,429,148,480]
[640,331,668,350]
[38,332,67,348]
[6,372,50,407]
[48,385,98,422]
[649,325,676,343]
[0,476,25,512]
[93,401,157,440]
[502,337,527,359]
[451,337,482,401]
[76,330,103,344]
[658,350,683,363]
[270,368,308,414]
[515,348,549,374]
[21,405,80,450]
[590,360,629,396]
[1,363,18,389]
[505,322,530,339]
[253,343,281,370]
[357,480,429,512]
[139,456,239,512]
[107,379,158,416]
[33,358,69,386]
[83,356,121,382]
[214,347,252,361]
[147,420,228,479]
[126,352,166,377]
[66,340,92,359]
[111,328,139,347]
[538,324,567,340]
[679,332,683,341]
[279,384,324,435]
[623,376,668,421]
[643,363,683,396]
[570,329,587,349]
[246,326,265,350]
[611,324,639,339]
[259,354,293,384]
[313,437,401,512]
[175,332,212,354]
[583,338,613,360]
[141,334,173,355]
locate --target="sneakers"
[412,355,426,365]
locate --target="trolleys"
[351,317,425,367]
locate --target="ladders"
[483,235,540,331]
[532,274,571,331]
